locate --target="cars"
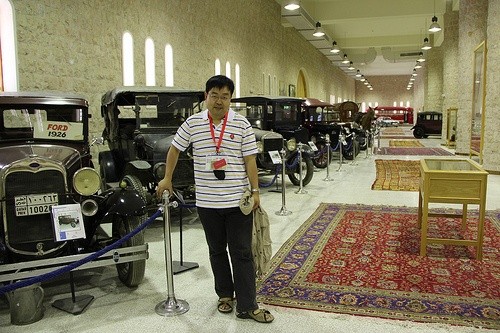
[409,111,442,139]
[229,94,333,169]
[97,88,208,231]
[251,129,314,187]
[333,101,374,151]
[0,90,149,300]
[301,97,360,160]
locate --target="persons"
[157,75,274,323]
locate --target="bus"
[365,104,414,125]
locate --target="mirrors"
[469,40,486,165]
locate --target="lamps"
[313,22,325,37]
[284,0,301,11]
[428,0,441,32]
[406,18,432,90]
[347,61,355,70]
[330,40,340,53]
[356,70,374,91]
[342,53,350,64]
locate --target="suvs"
[375,116,399,127]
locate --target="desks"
[417,159,489,261]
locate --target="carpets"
[389,140,426,148]
[382,128,413,132]
[383,132,405,135]
[375,135,415,139]
[371,159,421,191]
[373,147,455,156]
[255,203,500,330]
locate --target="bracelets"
[252,188,260,192]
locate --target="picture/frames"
[289,84,296,97]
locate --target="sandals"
[218,297,233,312]
[236,308,274,323]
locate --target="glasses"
[208,92,232,101]
